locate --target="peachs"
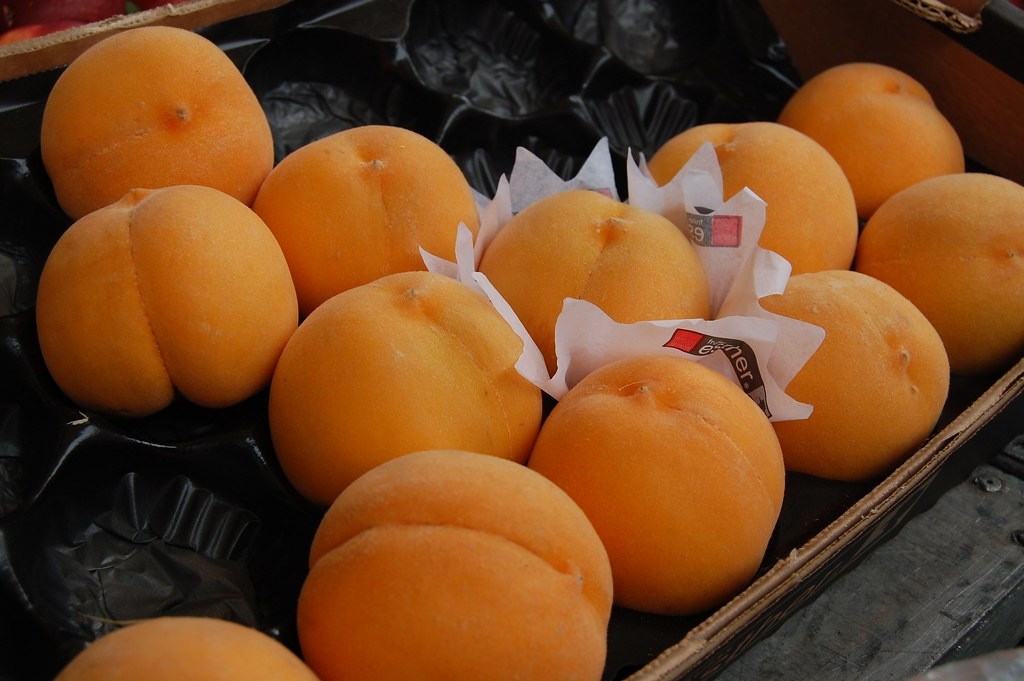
[38,25,1024,681]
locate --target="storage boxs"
[0,0,1024,681]
